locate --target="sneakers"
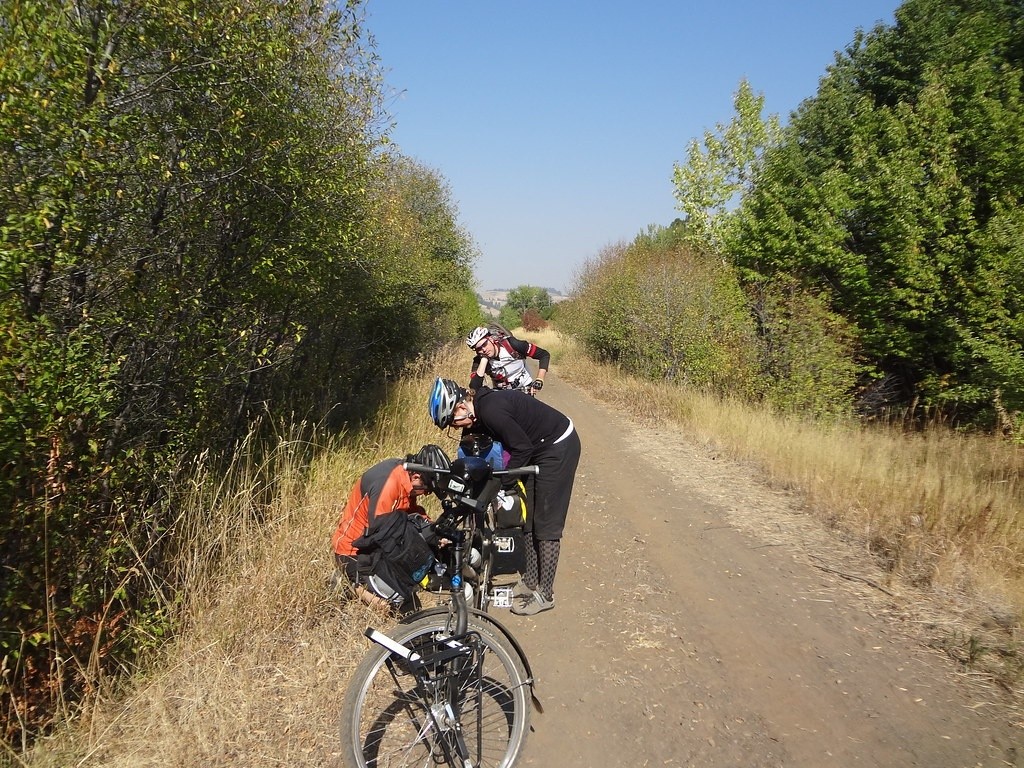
[327,568,344,599]
[498,579,539,598]
[510,587,555,615]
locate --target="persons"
[327,443,454,626]
[427,377,582,617]
[465,322,551,396]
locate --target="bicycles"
[338,380,544,768]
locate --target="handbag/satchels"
[496,477,528,528]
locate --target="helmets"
[465,326,489,349]
[411,444,451,500]
[428,376,461,431]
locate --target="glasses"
[475,338,489,351]
[449,401,462,427]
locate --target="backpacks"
[485,323,512,337]
[351,509,436,604]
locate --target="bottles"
[469,548,481,570]
[464,581,473,609]
